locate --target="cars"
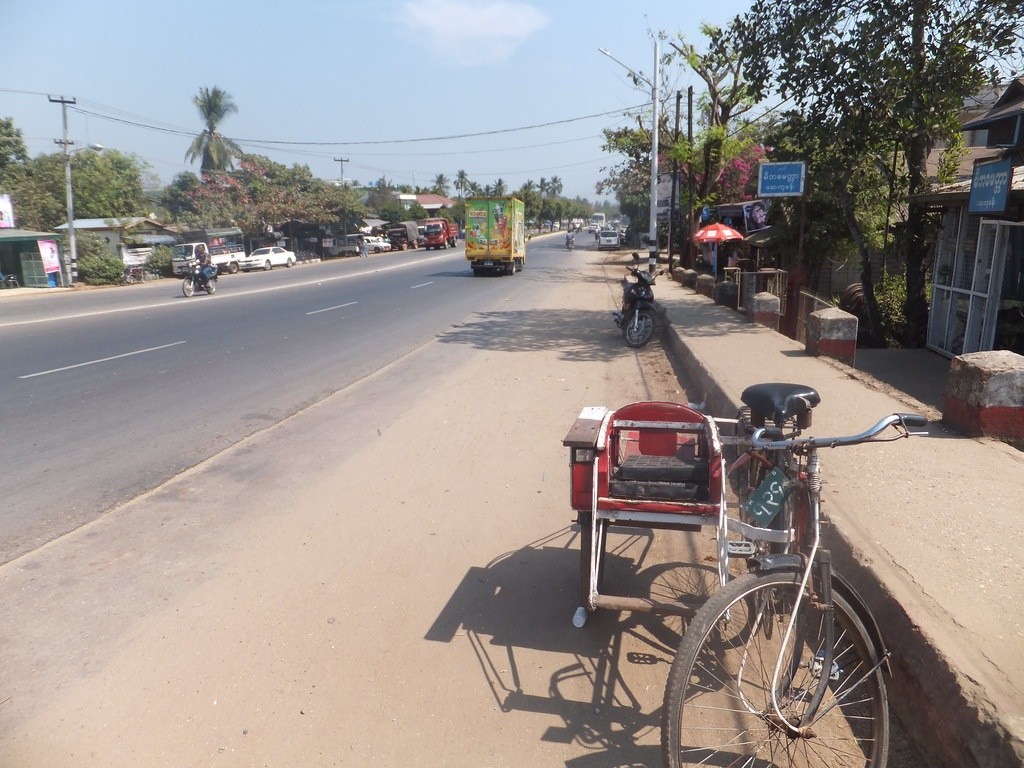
[598,231,620,249]
[607,219,629,244]
[238,246,296,273]
[524,218,584,233]
[364,236,392,254]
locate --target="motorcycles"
[612,252,664,347]
[181,257,217,297]
[565,234,575,252]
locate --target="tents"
[0,229,69,288]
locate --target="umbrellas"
[693,223,744,276]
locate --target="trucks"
[464,195,532,275]
[386,221,419,250]
[172,243,246,274]
[423,217,460,250]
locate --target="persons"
[361,239,368,257]
[748,201,771,230]
[528,231,531,242]
[566,230,576,249]
[594,222,618,243]
[186,244,213,288]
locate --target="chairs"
[608,401,711,503]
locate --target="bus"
[591,213,606,230]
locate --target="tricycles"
[564,384,929,768]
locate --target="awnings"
[123,234,177,245]
[362,219,390,228]
[182,227,244,240]
[744,226,790,249]
